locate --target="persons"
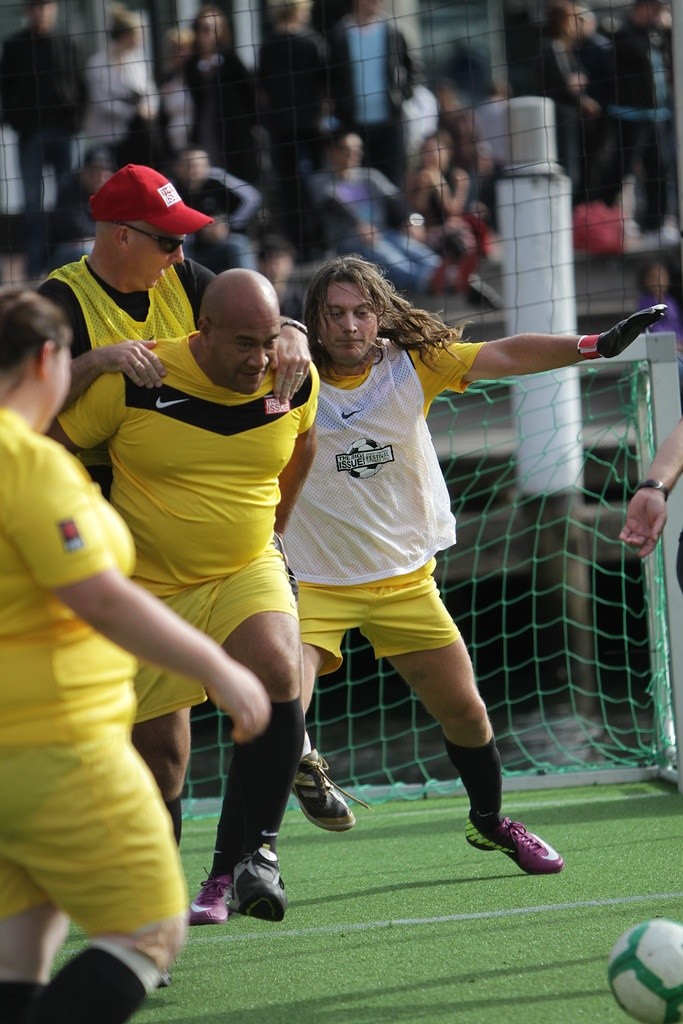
[620,412,683,599]
[37,163,357,922]
[189,255,669,924]
[0,1,680,286]
[1,288,274,1024]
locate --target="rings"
[297,371,304,376]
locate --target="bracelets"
[635,479,670,502]
[281,320,309,336]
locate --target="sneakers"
[189,865,233,924]
[464,808,563,874]
[225,842,285,922]
[290,749,375,832]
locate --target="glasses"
[113,221,185,254]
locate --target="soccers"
[606,918,683,1023]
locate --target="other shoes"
[158,970,171,988]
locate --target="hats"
[90,163,213,235]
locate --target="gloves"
[576,304,670,359]
